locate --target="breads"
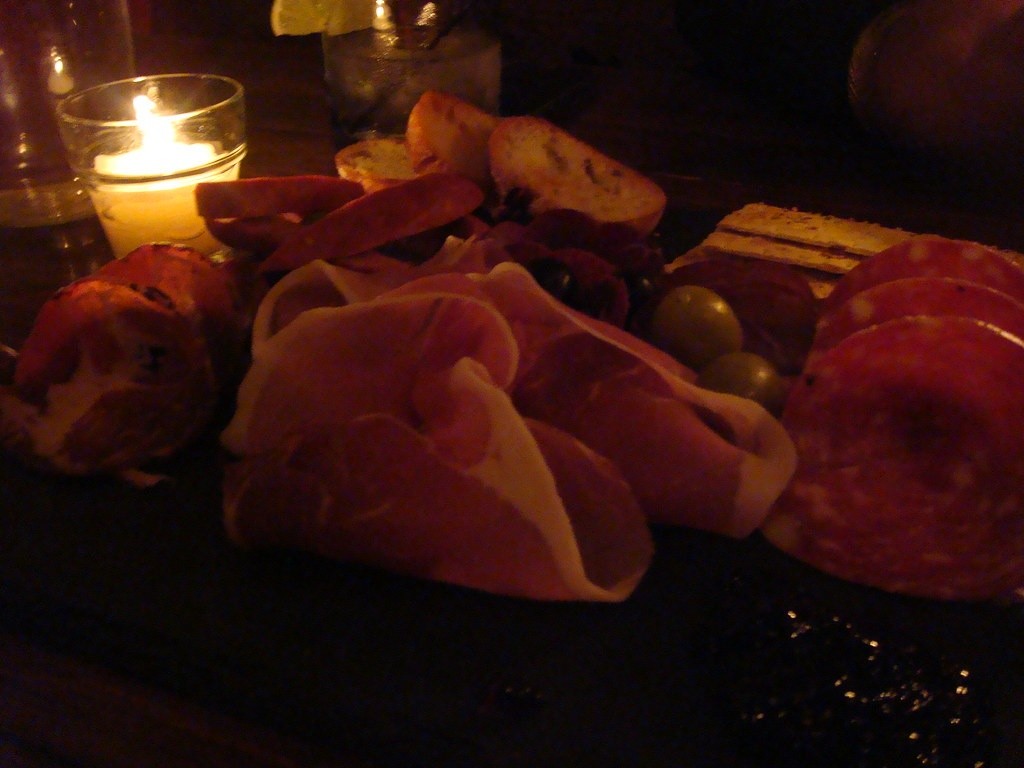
[196,89,667,271]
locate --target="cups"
[53,72,245,265]
[322,1,503,197]
[0,0,140,226]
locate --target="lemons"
[270,0,392,36]
